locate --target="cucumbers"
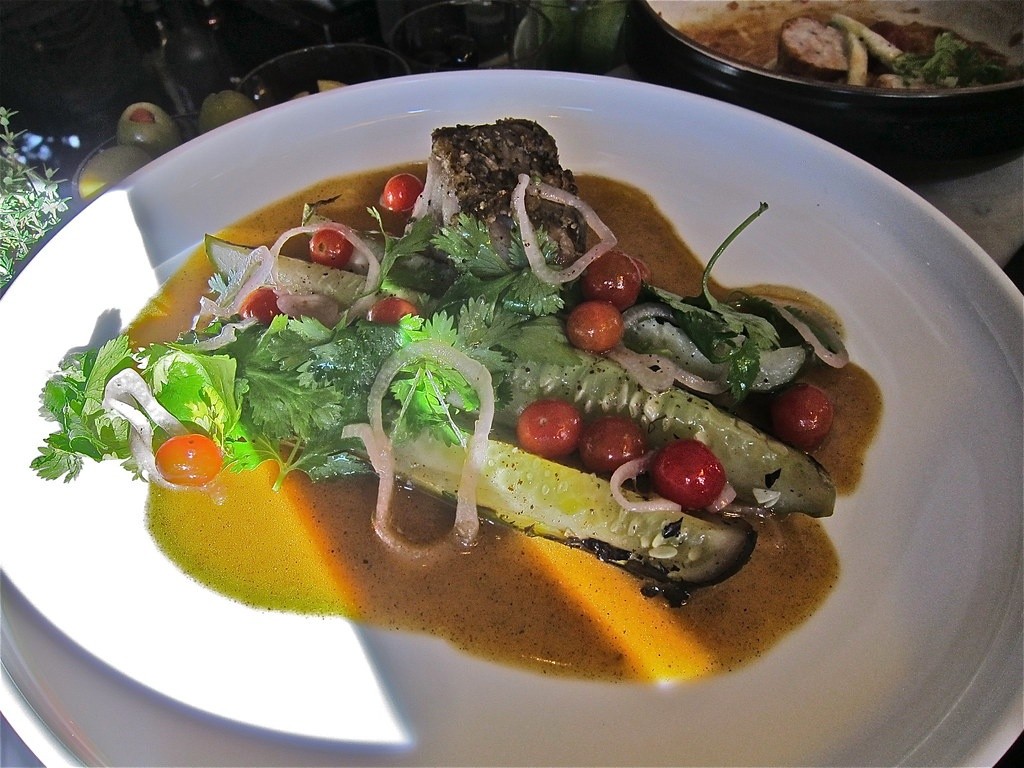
[393,343,837,587]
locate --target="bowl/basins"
[634,0,1024,185]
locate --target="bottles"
[141,0,233,112]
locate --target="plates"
[0,70,1024,768]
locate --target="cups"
[72,0,654,212]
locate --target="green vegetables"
[885,29,1024,88]
[30,202,832,492]
